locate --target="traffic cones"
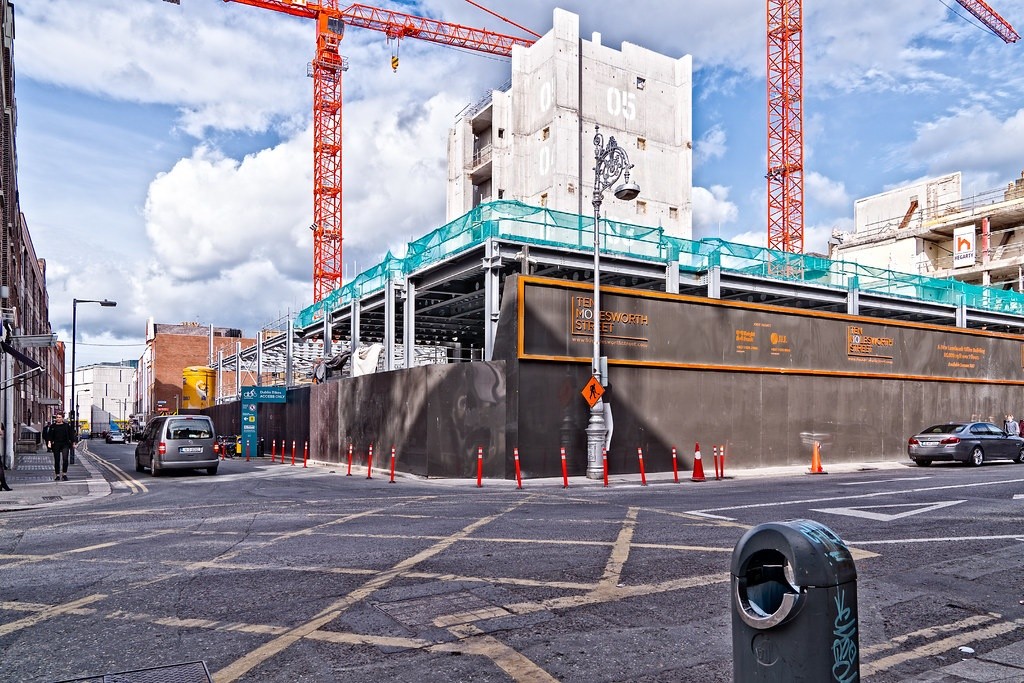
[805,441,828,474]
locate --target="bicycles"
[219,436,237,457]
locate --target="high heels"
[0,483,13,491]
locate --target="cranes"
[766,0,1021,278]
[165,0,542,306]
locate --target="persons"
[48,411,78,481]
[0,421,13,491]
[1003,414,1021,437]
[42,422,52,453]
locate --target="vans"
[134,415,219,477]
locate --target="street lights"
[70,299,117,464]
[76,389,90,443]
[586,121,641,479]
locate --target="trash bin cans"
[731,518,862,683]
[257,437,265,457]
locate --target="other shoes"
[55,476,60,481]
[62,474,68,481]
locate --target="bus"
[65,419,89,439]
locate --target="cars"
[106,431,125,444]
[908,421,1024,467]
